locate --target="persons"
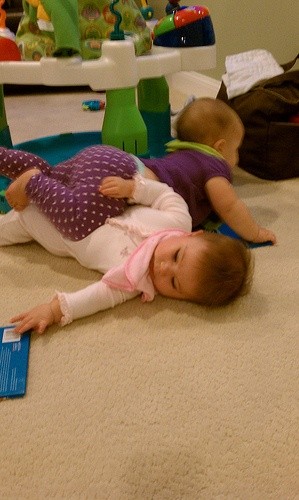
[0,94,278,246]
[0,174,255,336]
[22,0,86,61]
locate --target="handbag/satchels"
[217,53,299,180]
